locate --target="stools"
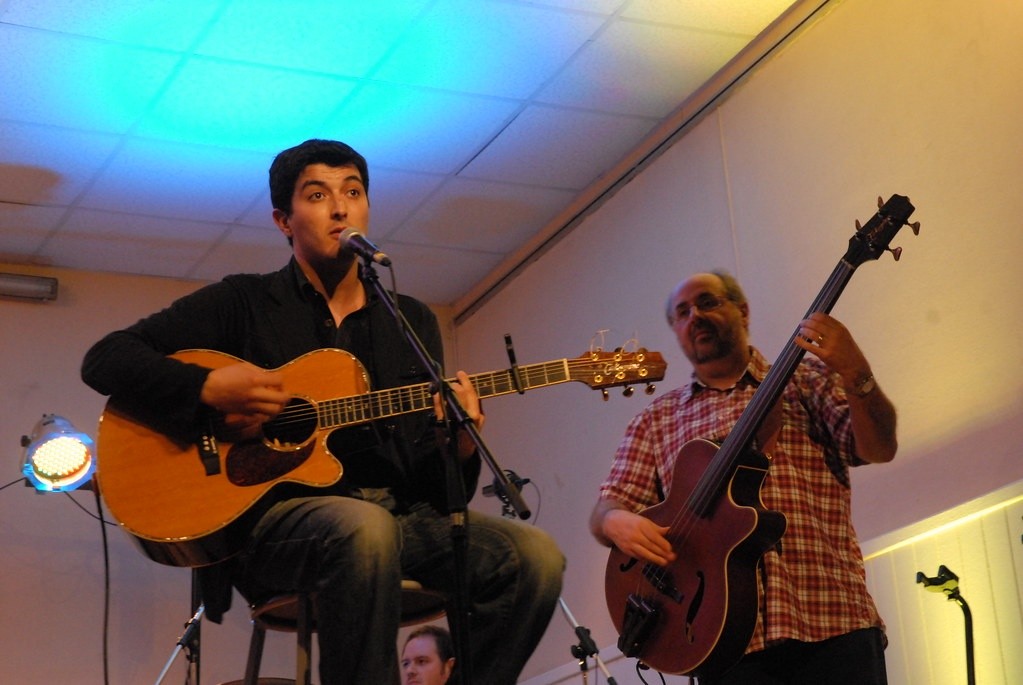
[244,579,447,685]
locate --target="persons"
[80,140,568,685]
[588,272,899,685]
[401,624,455,685]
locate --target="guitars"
[605,193,921,682]
[95,328,668,568]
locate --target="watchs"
[845,372,875,397]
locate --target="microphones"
[482,478,530,498]
[339,227,391,267]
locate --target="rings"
[816,334,824,343]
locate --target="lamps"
[18,412,112,685]
[0,272,58,303]
